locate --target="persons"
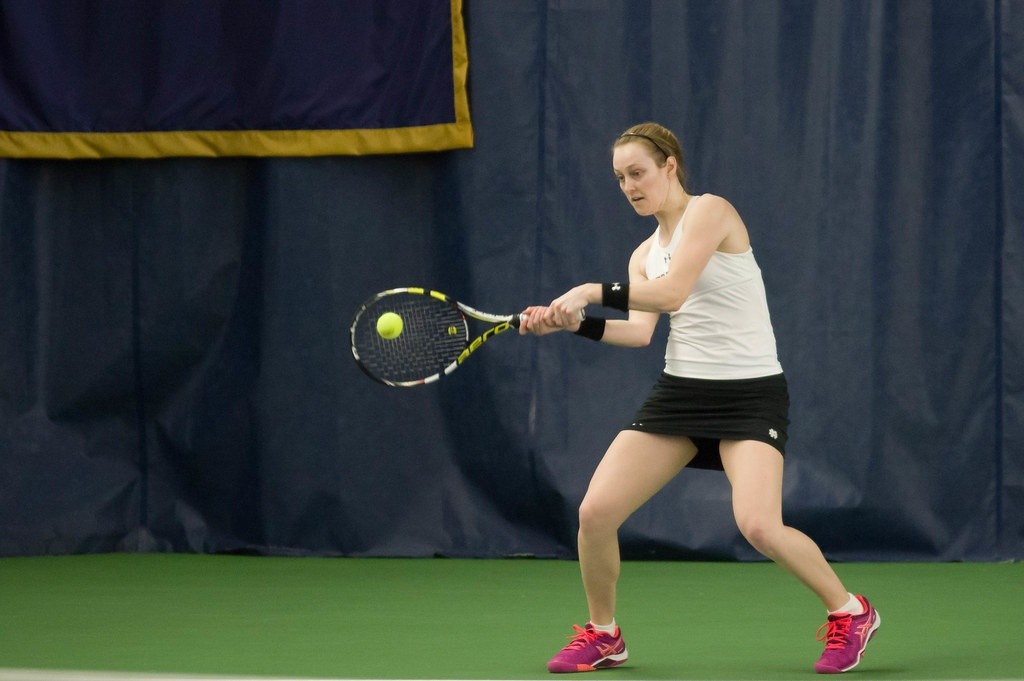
[520,124,881,673]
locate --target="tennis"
[375,312,404,340]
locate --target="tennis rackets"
[349,286,587,388]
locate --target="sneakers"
[814,594,881,674]
[547,622,628,672]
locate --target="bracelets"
[602,282,630,313]
[572,315,606,343]
